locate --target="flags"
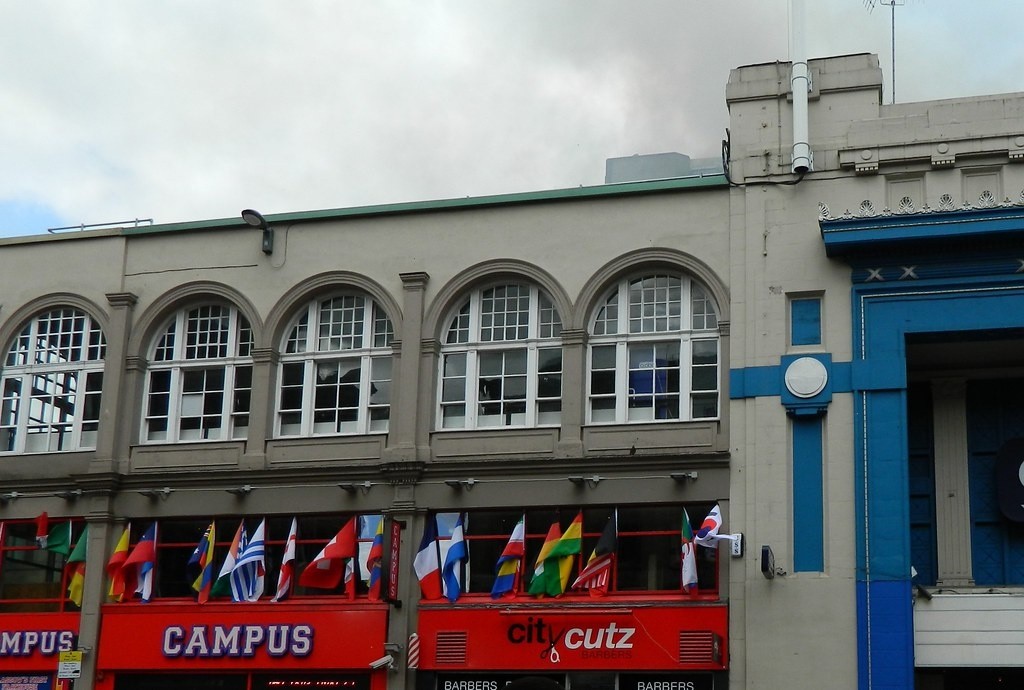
[35,504,723,603]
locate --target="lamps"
[242,208,277,256]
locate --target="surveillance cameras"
[916,584,933,601]
[368,654,396,672]
